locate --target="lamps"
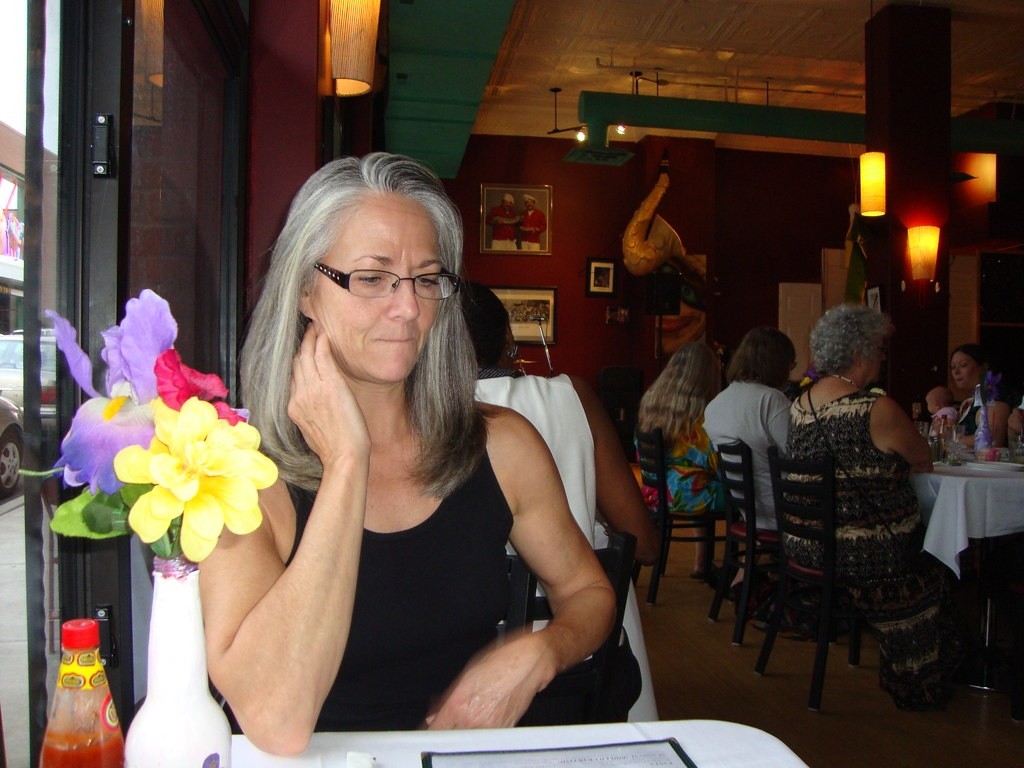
[908,226,940,280]
[132,0,165,129]
[859,152,887,217]
[316,0,381,99]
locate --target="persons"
[198,153,616,757]
[786,303,974,713]
[489,193,547,250]
[950,343,1010,449]
[926,387,957,439]
[633,342,724,581]
[459,279,661,569]
[1007,395,1024,447]
[704,327,795,629]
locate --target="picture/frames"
[866,282,885,313]
[486,283,559,347]
[584,254,619,300]
[479,182,554,257]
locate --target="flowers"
[32,286,280,574]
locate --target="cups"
[1009,434,1024,464]
[914,422,965,466]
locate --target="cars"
[0,395,24,498]
[0,328,61,470]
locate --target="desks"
[226,719,810,768]
[906,454,1024,724]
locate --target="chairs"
[628,420,890,712]
[487,528,638,728]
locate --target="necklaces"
[832,374,857,386]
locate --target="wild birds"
[930,397,975,440]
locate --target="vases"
[122,556,232,768]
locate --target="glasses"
[313,262,461,301]
[791,361,798,370]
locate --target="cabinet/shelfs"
[777,246,851,382]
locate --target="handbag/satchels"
[607,531,638,578]
[905,523,933,568]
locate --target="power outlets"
[605,302,630,326]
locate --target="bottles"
[939,414,951,462]
[974,405,992,462]
[39,618,125,768]
[124,554,233,768]
